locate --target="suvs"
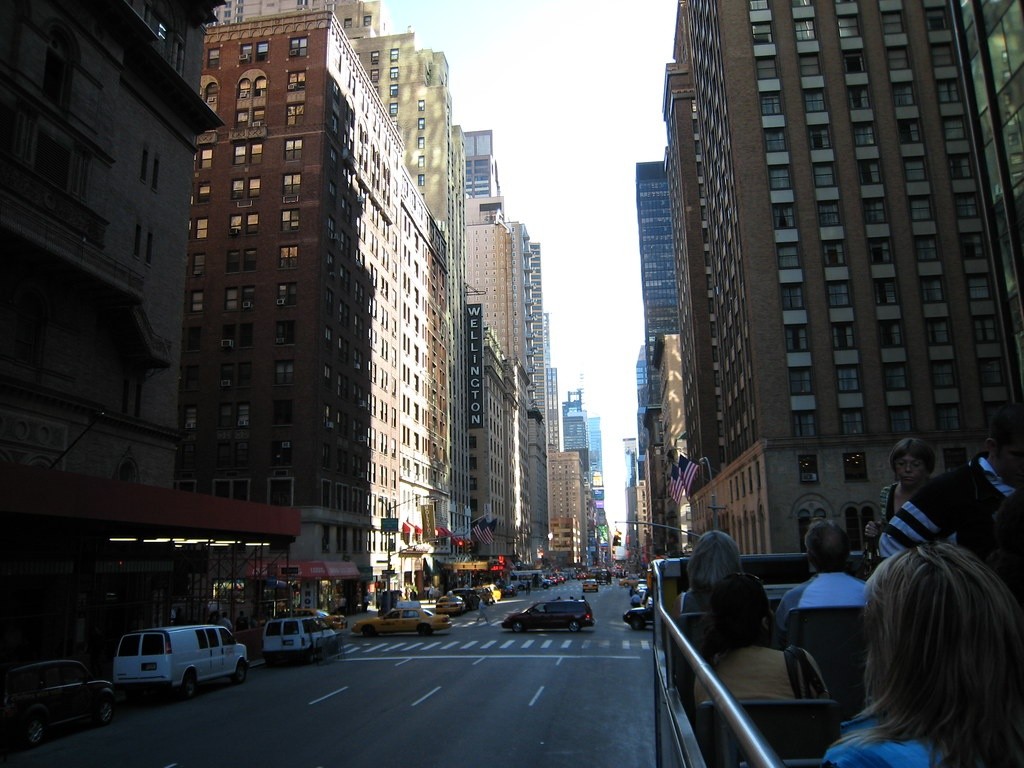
[501,595,595,633]
[262,617,338,669]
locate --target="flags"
[676,455,700,500]
[473,518,497,544]
[668,464,684,505]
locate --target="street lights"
[698,456,719,530]
[387,496,434,612]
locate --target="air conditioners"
[236,442,247,450]
[238,54,250,62]
[221,339,234,347]
[326,421,334,429]
[237,419,249,427]
[220,379,232,387]
[357,400,368,408]
[288,84,295,90]
[358,195,364,204]
[240,93,247,98]
[242,300,251,308]
[207,96,215,101]
[276,298,286,305]
[275,337,286,344]
[194,271,202,275]
[252,122,261,127]
[289,50,298,56]
[185,421,196,429]
[228,228,238,235]
[331,232,340,241]
[359,435,367,442]
[282,441,291,447]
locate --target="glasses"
[723,572,763,609]
[894,460,927,471]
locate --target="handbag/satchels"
[855,521,881,581]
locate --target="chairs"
[673,604,866,768]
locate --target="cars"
[473,584,501,603]
[623,607,654,631]
[549,569,612,586]
[0,660,117,748]
[453,588,480,610]
[501,584,519,598]
[619,577,648,592]
[436,590,466,617]
[582,579,598,592]
[293,609,348,630]
[351,601,452,637]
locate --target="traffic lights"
[613,531,622,546]
[458,539,471,553]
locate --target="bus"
[510,570,552,592]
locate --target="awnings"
[451,536,473,546]
[403,522,422,534]
[435,527,451,537]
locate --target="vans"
[112,624,250,704]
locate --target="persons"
[412,568,641,624]
[670,398,1024,768]
[207,610,251,633]
